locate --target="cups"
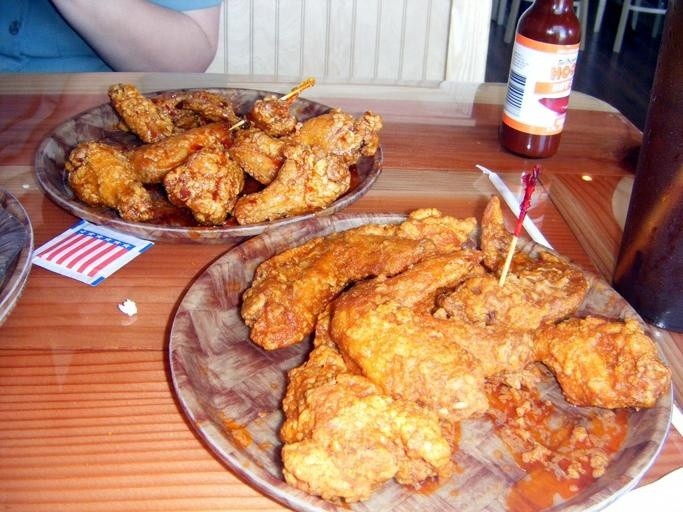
[606,1,683,337]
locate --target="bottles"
[494,0,583,160]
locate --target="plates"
[165,207,674,512]
[31,84,385,249]
[0,185,37,327]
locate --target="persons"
[1,0,222,75]
